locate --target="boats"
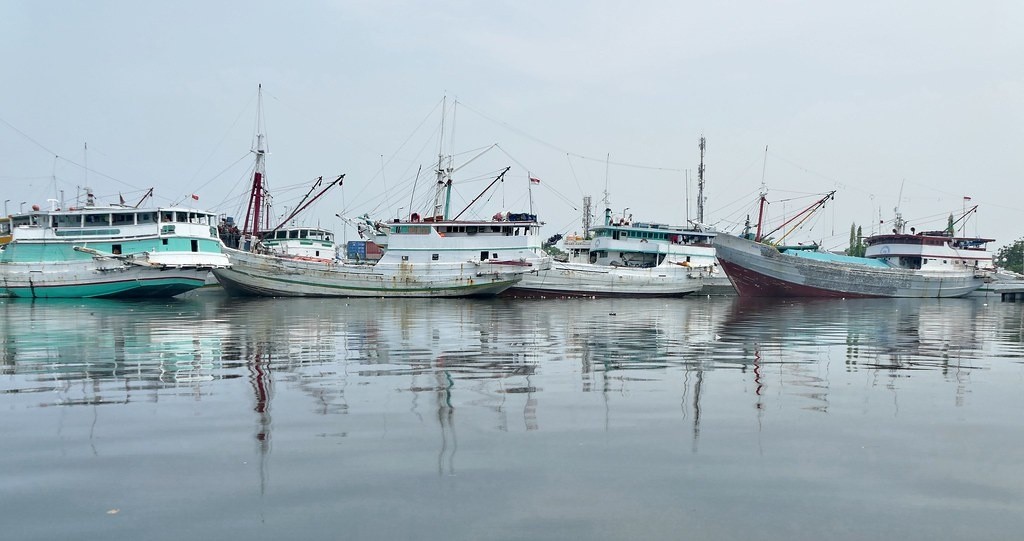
[688,186,986,300]
[0,186,230,299]
[504,253,715,299]
[208,84,552,298]
[585,140,1024,295]
